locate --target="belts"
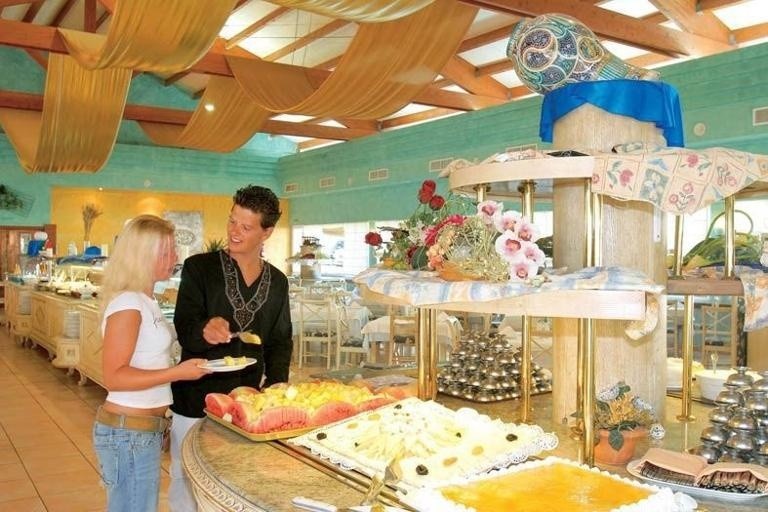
[95,406,173,433]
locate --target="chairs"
[287,277,436,371]
[666,300,739,370]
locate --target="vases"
[595,427,649,466]
[84,241,90,253]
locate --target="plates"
[626,459,768,500]
[197,358,258,373]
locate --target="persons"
[93,217,212,511]
[168,183,291,511]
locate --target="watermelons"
[227,402,258,431]
[228,386,260,398]
[203,393,232,419]
[310,400,356,427]
[373,386,406,400]
[359,398,394,410]
[253,407,310,433]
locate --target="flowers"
[566,377,667,453]
[361,178,547,285]
[81,202,104,240]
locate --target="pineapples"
[245,382,376,413]
[222,355,249,368]
[238,331,262,346]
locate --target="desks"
[364,315,460,369]
[3,279,108,392]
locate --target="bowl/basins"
[694,369,738,401]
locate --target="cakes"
[313,396,528,483]
[422,454,678,512]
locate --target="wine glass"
[436,332,553,403]
[697,368,768,466]
